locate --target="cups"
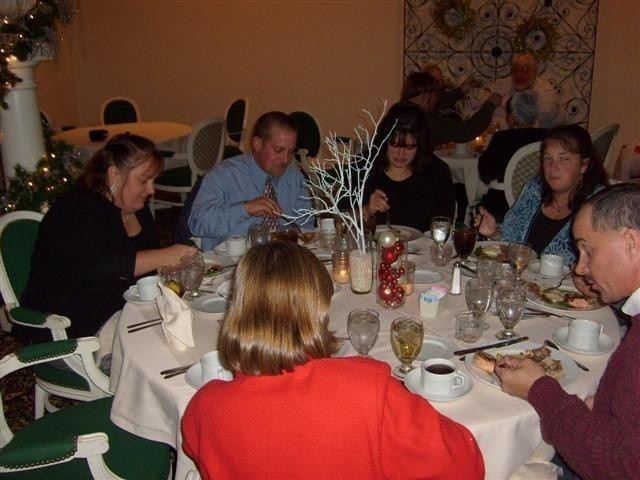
[218,236,246,256]
[420,358,465,396]
[275,224,299,244]
[128,276,160,301]
[200,350,234,385]
[539,254,564,276]
[319,217,336,233]
[376,233,408,310]
[567,318,604,351]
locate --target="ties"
[262,177,279,232]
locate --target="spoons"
[543,339,590,372]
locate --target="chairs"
[0,335,177,480]
[586,119,620,168]
[287,107,359,217]
[502,135,546,207]
[1,210,148,421]
[151,116,226,223]
[98,96,139,128]
[219,94,249,161]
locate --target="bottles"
[449,261,463,296]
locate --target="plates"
[412,270,442,285]
[315,239,333,253]
[190,293,230,314]
[415,336,456,363]
[458,241,537,272]
[212,241,254,256]
[122,289,157,305]
[403,367,473,402]
[520,277,610,313]
[424,230,432,240]
[374,224,424,243]
[463,341,577,391]
[184,361,204,391]
[552,326,613,355]
[525,260,572,279]
[216,279,232,298]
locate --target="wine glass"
[430,244,451,267]
[159,264,182,297]
[390,316,424,379]
[497,273,516,297]
[356,214,377,238]
[495,286,525,341]
[247,222,271,245]
[346,307,380,357]
[509,241,531,280]
[476,255,498,288]
[464,278,495,330]
[452,230,476,266]
[431,222,450,245]
[179,254,205,302]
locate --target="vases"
[349,227,374,296]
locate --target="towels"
[155,280,195,352]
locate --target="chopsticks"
[127,318,162,334]
[453,336,529,356]
[160,364,193,379]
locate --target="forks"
[384,189,392,229]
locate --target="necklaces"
[121,215,130,225]
[551,203,567,218]
[552,194,570,213]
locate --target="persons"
[474,124,609,271]
[180,242,485,480]
[186,110,315,253]
[496,181,640,480]
[12,132,196,348]
[422,62,457,110]
[341,107,454,233]
[501,51,566,127]
[378,71,500,148]
